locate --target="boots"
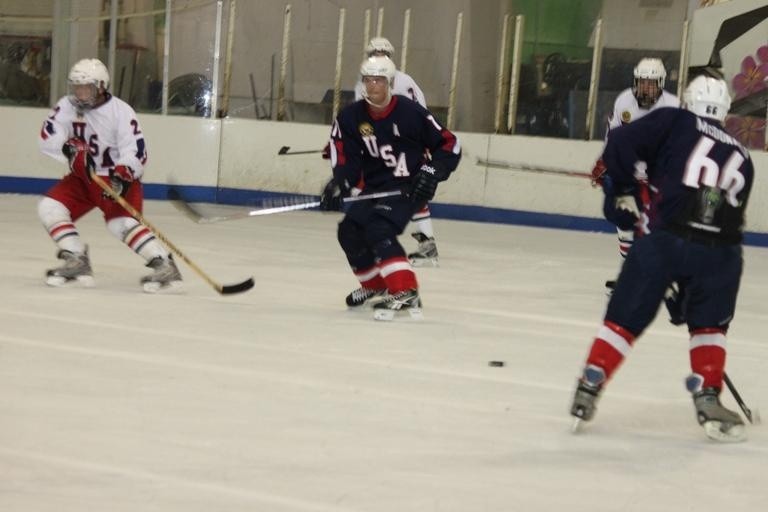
[693,388,747,444]
[139,253,188,296]
[605,281,616,297]
[570,376,601,435]
[45,245,91,287]
[373,290,425,322]
[346,285,388,312]
[408,232,441,267]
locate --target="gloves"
[318,177,349,212]
[666,290,688,327]
[102,165,135,202]
[401,166,438,212]
[604,185,645,231]
[62,135,96,185]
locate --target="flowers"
[724,114,765,150]
[730,55,768,101]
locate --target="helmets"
[68,58,110,90]
[360,56,395,90]
[366,37,395,57]
[633,57,667,89]
[681,74,731,123]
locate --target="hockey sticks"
[89,166,254,296]
[165,187,405,226]
[278,145,327,156]
[634,212,768,424]
[475,160,598,178]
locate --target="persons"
[321,36,439,258]
[570,73,754,424]
[37,57,183,283]
[606,57,683,291]
[321,56,460,309]
